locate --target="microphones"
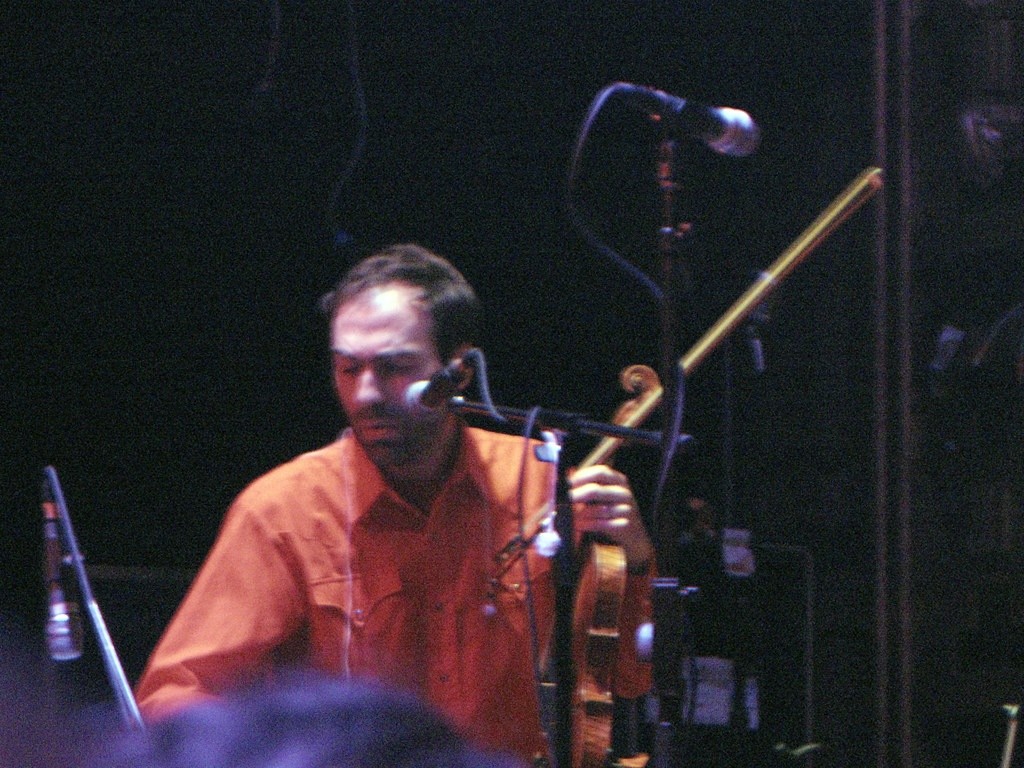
[42,480,83,660]
[618,82,757,156]
[407,352,477,422]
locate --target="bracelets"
[623,548,658,577]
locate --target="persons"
[132,244,661,768]
[676,497,719,549]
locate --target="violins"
[553,363,662,768]
